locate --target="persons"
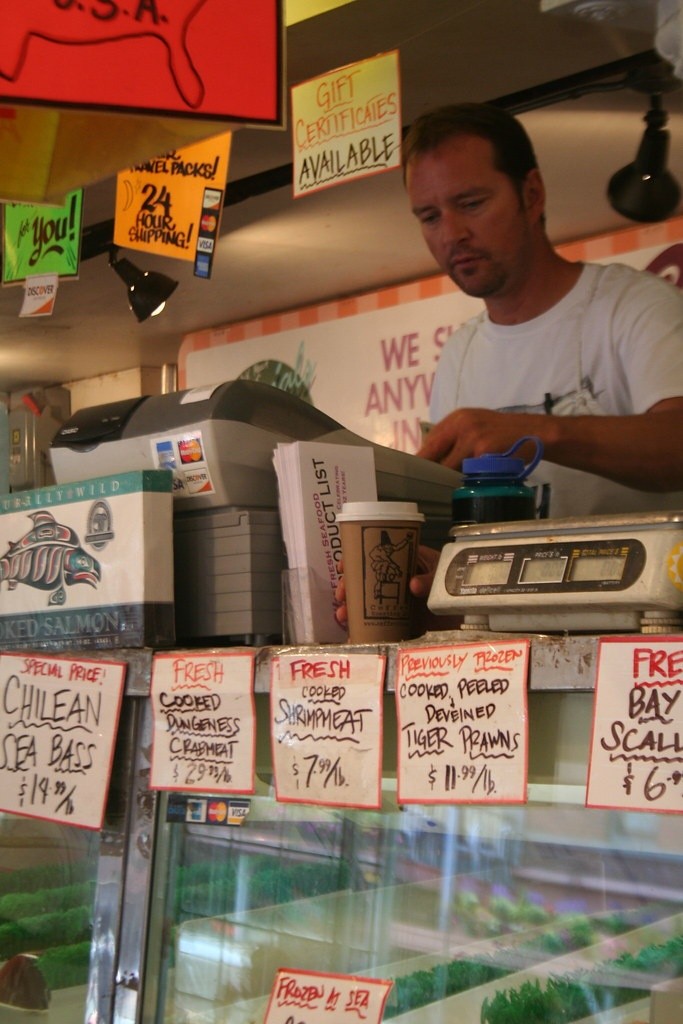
[401,104,683,517]
[334,543,451,626]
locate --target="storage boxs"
[0,464,175,658]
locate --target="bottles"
[450,435,543,543]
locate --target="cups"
[335,501,425,645]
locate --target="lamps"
[605,94,680,221]
[108,240,179,325]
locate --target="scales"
[427,509,683,636]
